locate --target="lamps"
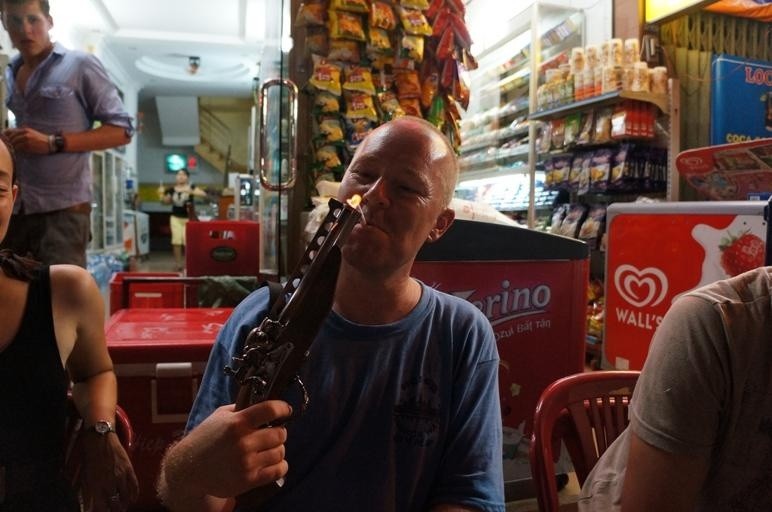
[527,371,646,511]
[68,389,138,466]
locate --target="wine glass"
[104,492,120,504]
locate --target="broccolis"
[89,149,125,254]
[605,202,767,375]
[412,216,591,498]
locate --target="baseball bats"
[223,196,366,490]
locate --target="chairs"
[68,389,138,466]
[527,371,646,511]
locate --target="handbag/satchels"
[48,133,67,152]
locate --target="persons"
[158,169,207,269]
[156,113,511,512]
[620,264,772,511]
[0,0,136,269]
[0,138,143,511]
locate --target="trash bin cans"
[86,419,116,437]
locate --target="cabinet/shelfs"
[453,0,681,365]
